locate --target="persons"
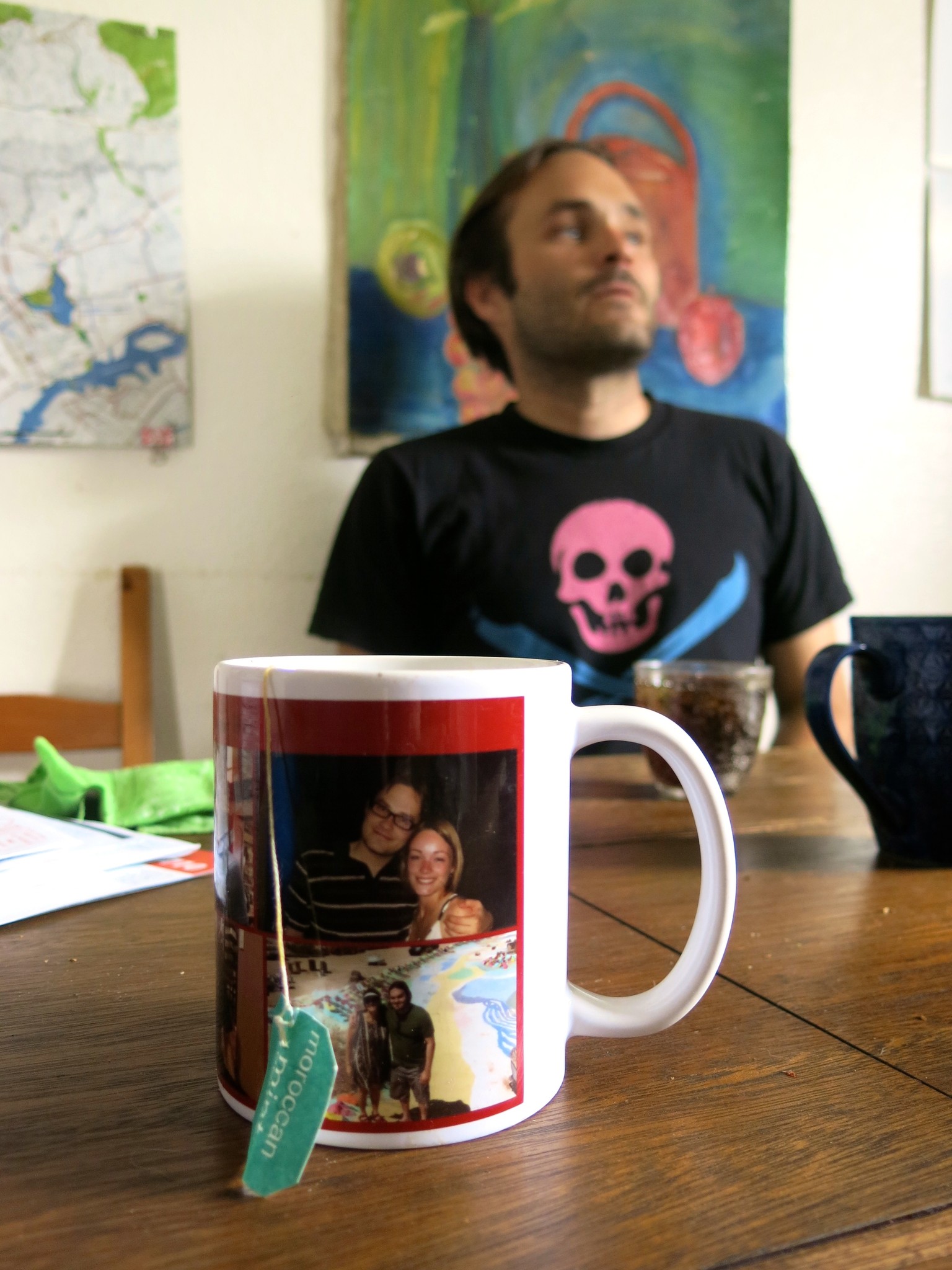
[346,980,435,1121]
[282,767,494,941]
[310,142,854,758]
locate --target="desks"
[0,742,952,1270]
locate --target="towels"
[0,735,216,835]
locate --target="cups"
[802,616,952,871]
[213,654,738,1150]
[635,659,774,799]
